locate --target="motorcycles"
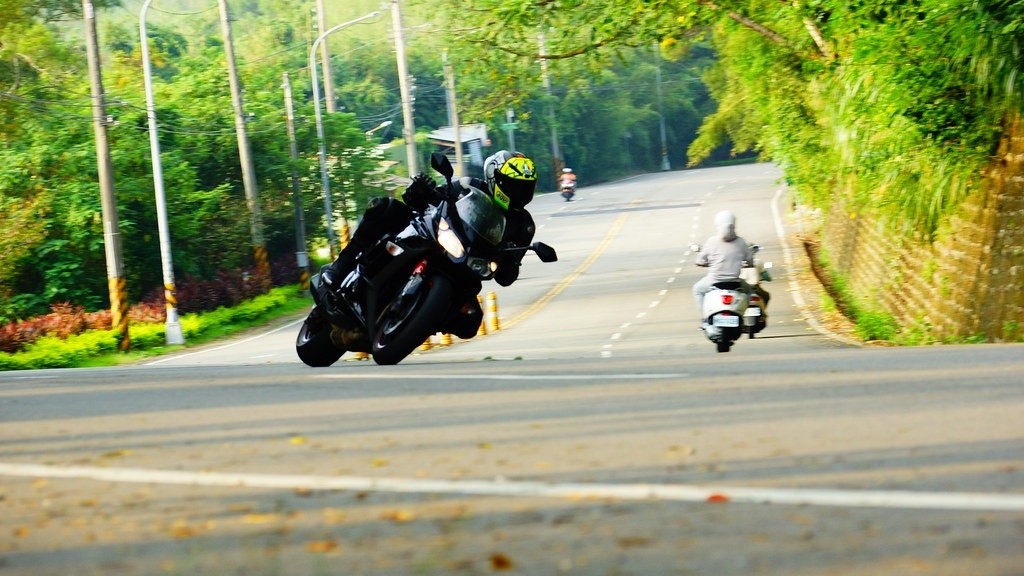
[688,241,773,353]
[561,181,575,200]
[294,148,558,372]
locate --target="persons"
[739,243,773,310]
[558,168,576,190]
[322,150,537,290]
[692,210,752,330]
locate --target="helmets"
[483,150,536,209]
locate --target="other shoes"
[322,264,344,288]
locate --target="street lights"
[366,120,393,144]
[311,12,382,259]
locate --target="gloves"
[407,172,437,199]
[498,241,523,263]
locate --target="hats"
[715,209,736,231]
[562,167,572,173]
